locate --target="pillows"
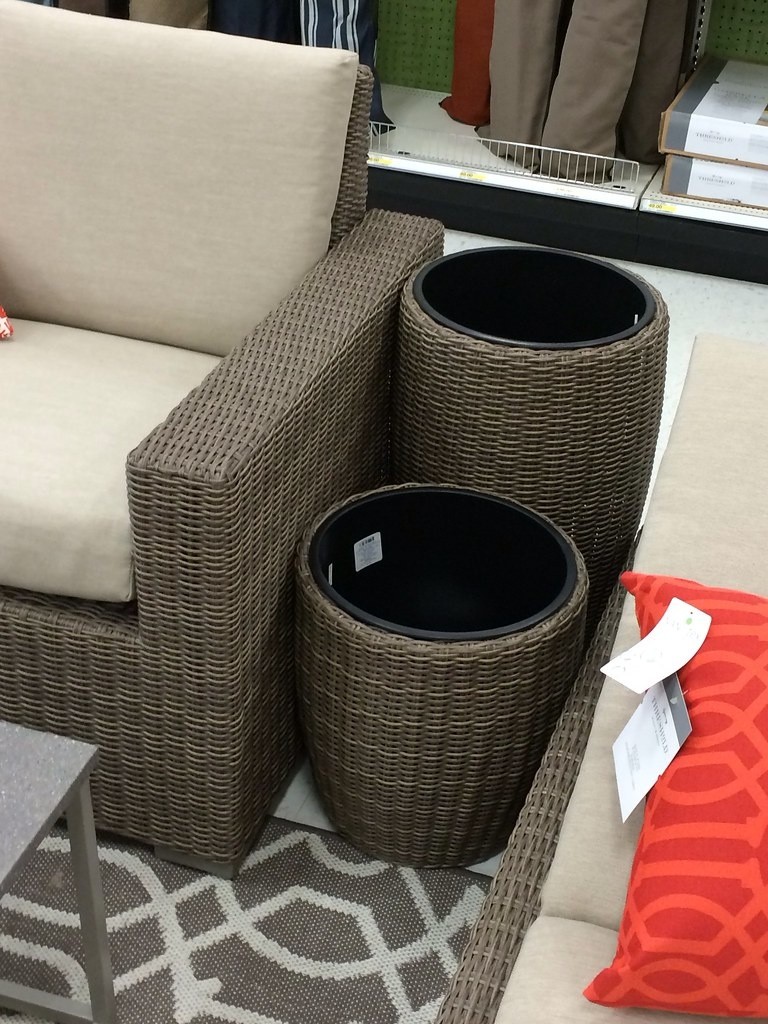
[584,572,768,1019]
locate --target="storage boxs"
[657,56,767,207]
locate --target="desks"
[1,719,118,1024]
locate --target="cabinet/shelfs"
[368,0,768,288]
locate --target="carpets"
[0,812,495,1024]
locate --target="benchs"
[430,334,767,1024]
[0,0,446,877]
